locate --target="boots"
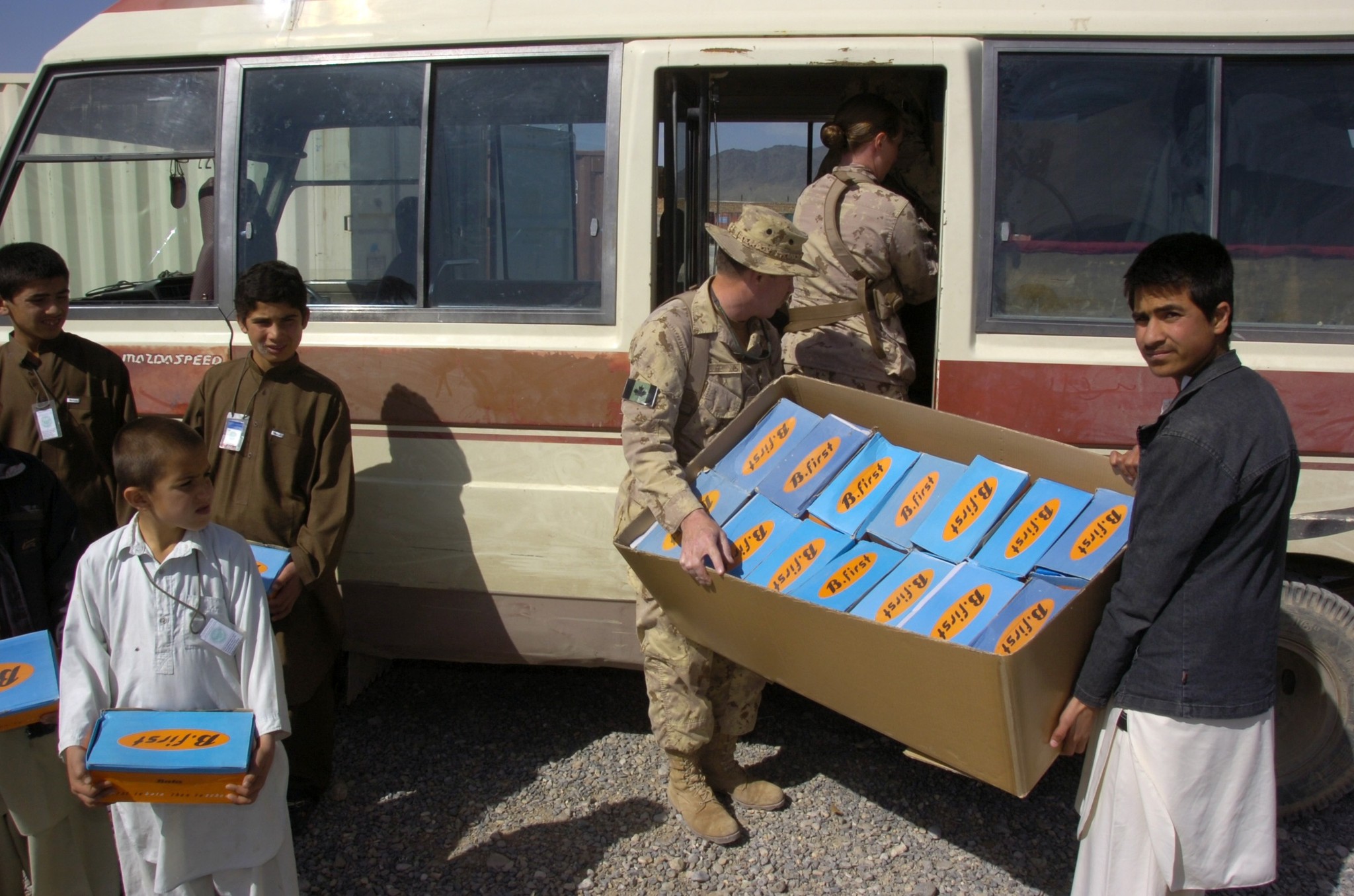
[696,735,785,811]
[666,749,741,844]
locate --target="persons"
[0,241,141,896]
[778,93,939,415]
[1048,233,1301,895]
[59,409,299,896]
[612,202,821,847]
[180,261,356,808]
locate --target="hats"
[705,206,820,278]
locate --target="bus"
[0,3,1354,829]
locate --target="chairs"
[189,176,277,304]
[373,196,417,304]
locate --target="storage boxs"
[246,539,292,636]
[0,629,60,732]
[84,707,254,806]
[614,374,1139,798]
